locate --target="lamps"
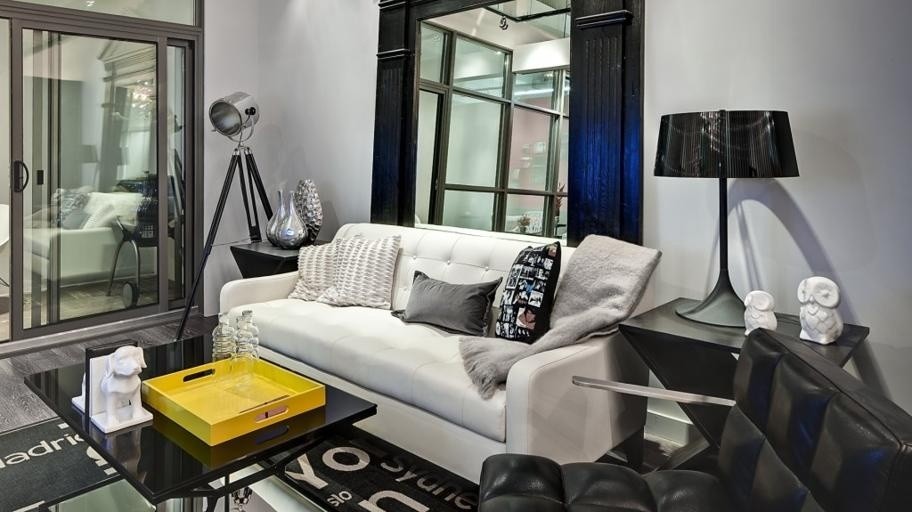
[652,109,801,328]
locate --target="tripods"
[173,146,273,344]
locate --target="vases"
[265,178,324,249]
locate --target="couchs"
[219,221,663,486]
[21,189,156,289]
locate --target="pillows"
[62,210,88,230]
[492,239,563,345]
[317,236,404,311]
[288,244,335,300]
[391,270,503,336]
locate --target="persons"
[519,308,537,330]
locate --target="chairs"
[478,329,911,510]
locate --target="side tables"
[617,295,869,472]
[230,239,333,278]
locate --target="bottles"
[265,190,288,246]
[211,308,259,395]
[276,190,309,251]
